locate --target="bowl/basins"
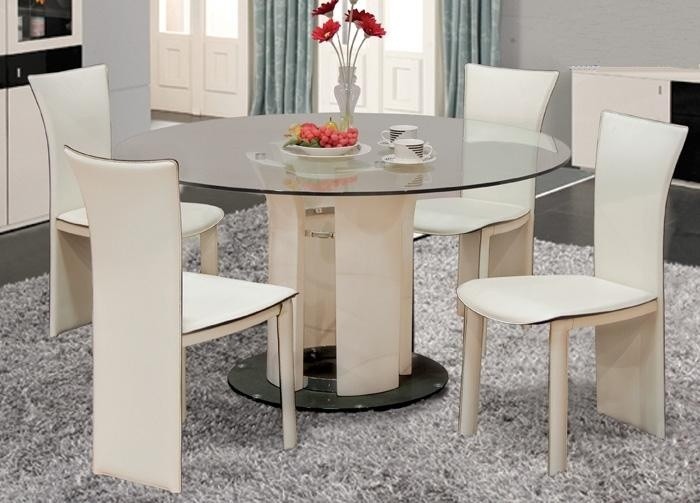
[290,141,360,155]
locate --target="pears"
[324,117,337,130]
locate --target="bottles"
[28,0,46,37]
[19,4,22,41]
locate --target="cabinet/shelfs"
[1,0,83,234]
[569,62,700,190]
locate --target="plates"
[380,153,435,168]
[281,143,372,158]
[379,139,395,148]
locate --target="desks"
[113,110,575,416]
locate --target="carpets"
[0,198,699,503]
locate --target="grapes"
[301,127,358,148]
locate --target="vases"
[335,66,362,120]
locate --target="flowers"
[308,0,387,110]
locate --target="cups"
[395,138,432,160]
[381,123,417,143]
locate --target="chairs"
[28,64,226,337]
[456,108,691,476]
[64,143,303,493]
[410,59,560,337]
